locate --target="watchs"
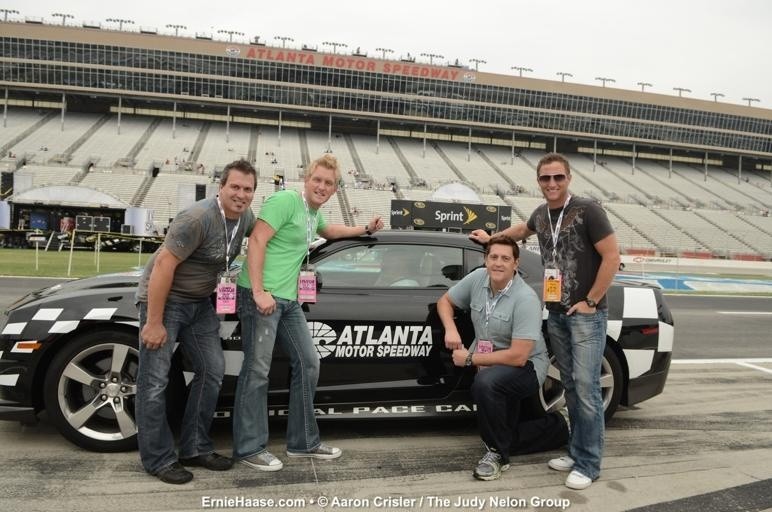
[464,353,473,368]
[585,298,598,307]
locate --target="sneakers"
[237,449,284,471]
[178,453,233,471]
[156,462,193,483]
[474,448,509,480]
[565,470,593,489]
[546,455,575,471]
[286,444,342,459]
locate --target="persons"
[232,155,383,473]
[469,152,620,490]
[135,160,259,484]
[17,208,25,230]
[436,235,571,481]
[380,245,421,288]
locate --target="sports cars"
[0,228,675,454]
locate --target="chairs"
[419,255,440,286]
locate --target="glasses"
[537,172,568,183]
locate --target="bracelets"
[365,225,372,236]
